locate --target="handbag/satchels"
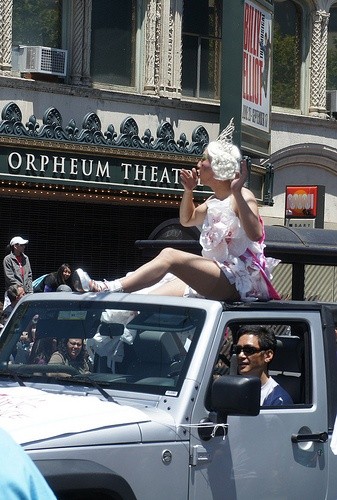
[32,273,52,292]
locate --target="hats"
[9,236,30,246]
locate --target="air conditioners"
[22,46,67,81]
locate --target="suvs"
[0,291,336,499]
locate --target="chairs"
[231,335,304,405]
[125,329,188,384]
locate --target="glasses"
[229,343,267,357]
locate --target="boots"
[72,268,124,295]
[92,337,124,363]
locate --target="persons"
[0,237,293,500]
[72,141,280,374]
